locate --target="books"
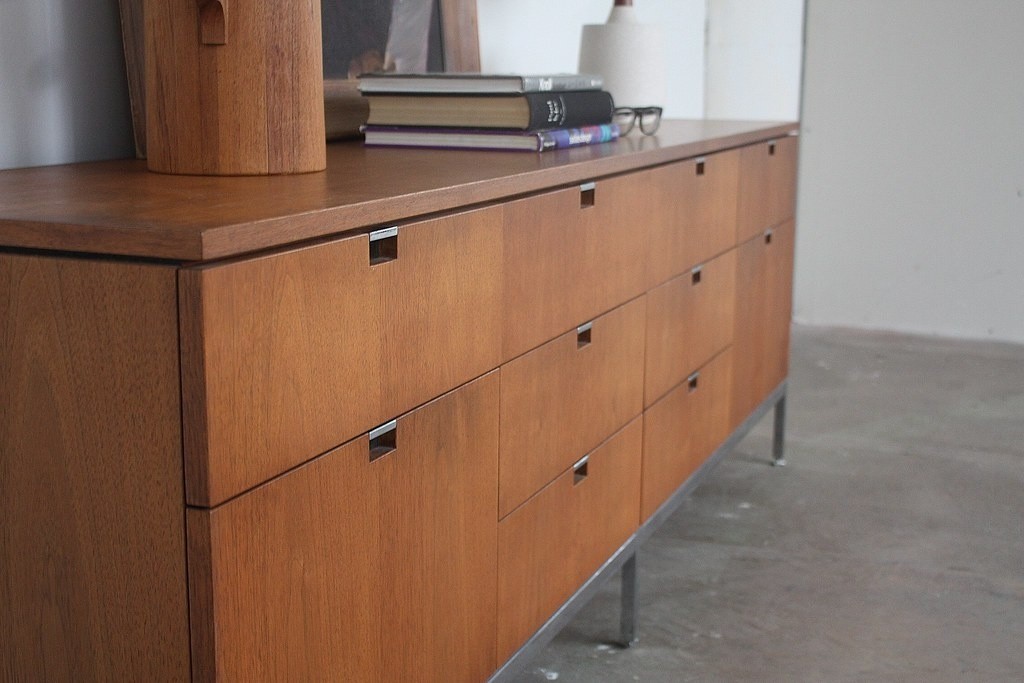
[353,67,620,151]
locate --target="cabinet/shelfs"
[0,119,802,683]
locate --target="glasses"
[610,107,662,136]
